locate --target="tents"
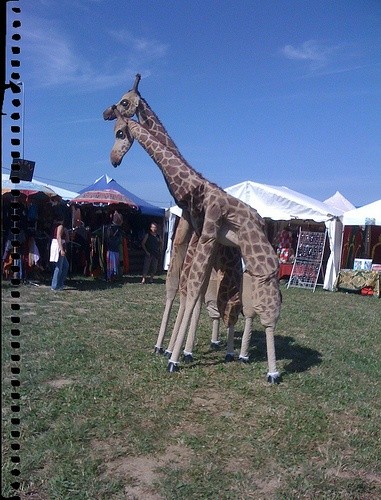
[164,179,381,298]
[1,171,166,271]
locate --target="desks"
[278,256,381,298]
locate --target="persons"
[49,212,70,292]
[141,222,163,283]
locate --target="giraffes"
[108,105,284,386]
[102,74,241,364]
